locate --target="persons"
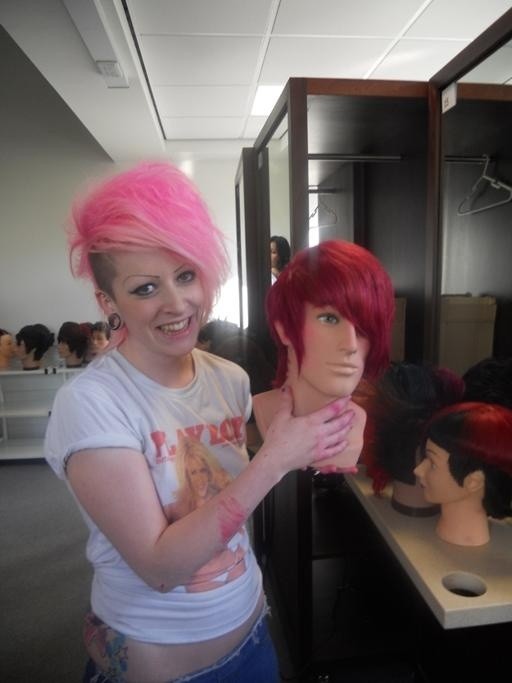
[409,396,512,547]
[263,232,293,286]
[79,321,96,363]
[38,150,358,680]
[161,430,250,597]
[251,238,397,471]
[14,321,54,371]
[56,319,87,368]
[90,319,111,355]
[377,354,477,516]
[0,326,16,370]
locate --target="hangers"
[306,186,338,228]
[459,152,512,216]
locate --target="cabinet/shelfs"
[0,363,86,462]
[234,78,442,681]
